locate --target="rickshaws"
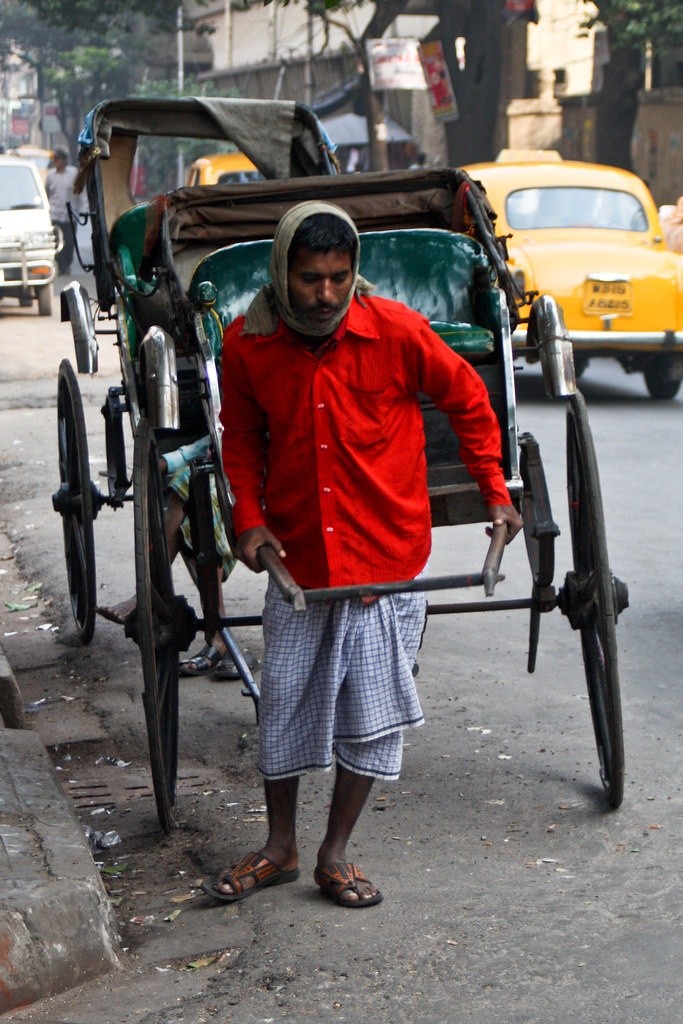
[52,94,352,720]
[104,170,636,842]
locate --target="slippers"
[212,652,264,679]
[177,645,226,678]
[200,850,300,901]
[314,864,383,907]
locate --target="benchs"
[190,228,492,355]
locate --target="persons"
[92,201,523,908]
[44,148,81,276]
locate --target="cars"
[0,154,64,316]
[184,150,268,189]
[6,142,58,188]
[449,147,682,400]
